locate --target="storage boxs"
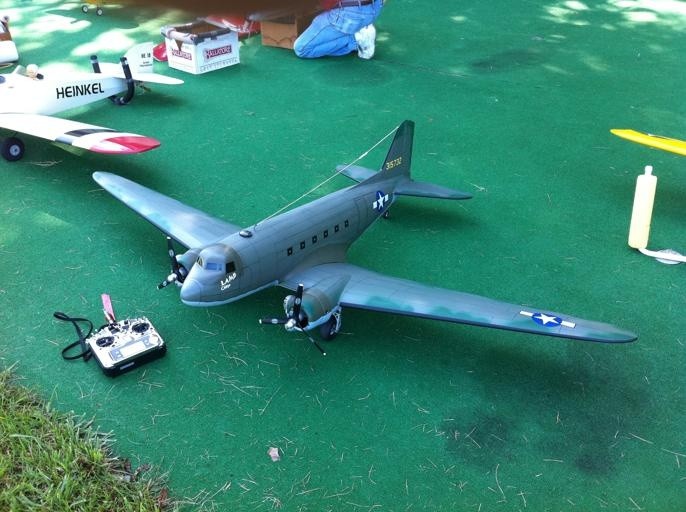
[260,15,313,50]
[160,20,240,76]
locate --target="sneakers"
[354,24,377,60]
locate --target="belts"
[332,0,377,9]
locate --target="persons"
[293,0,383,60]
[27,63,39,81]
